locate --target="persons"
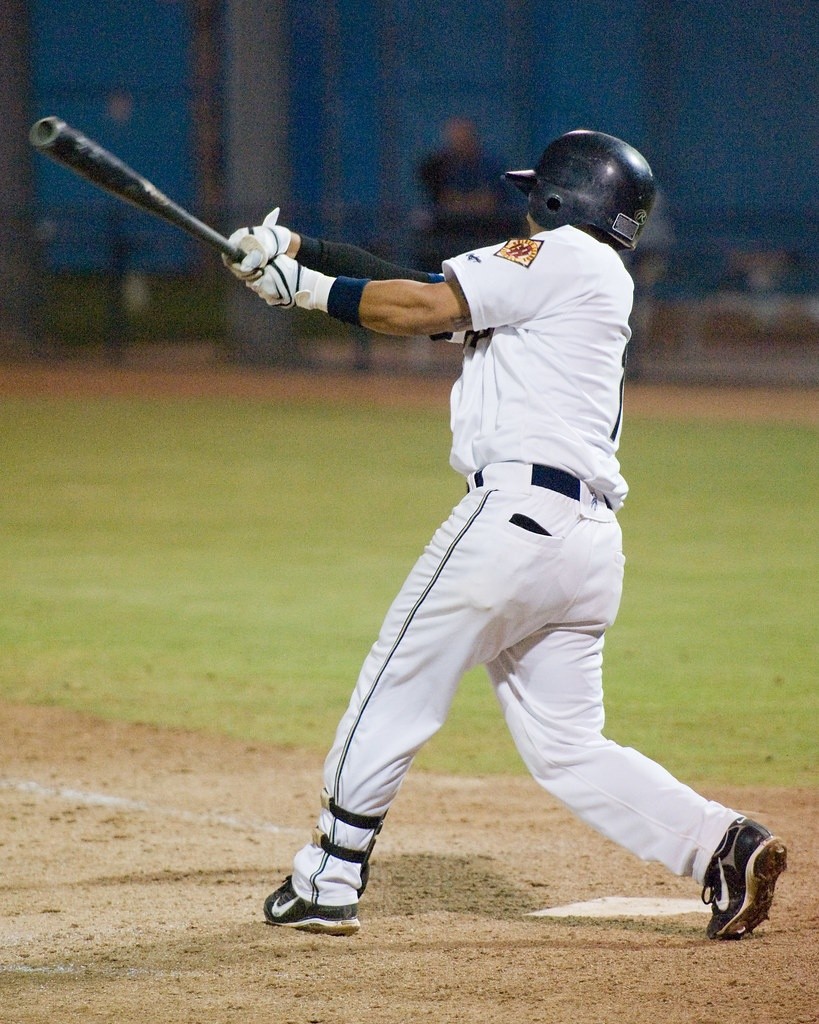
[223,126,788,941]
[403,117,505,328]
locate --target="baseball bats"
[27,115,243,258]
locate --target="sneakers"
[701,817,787,940]
[264,875,361,935]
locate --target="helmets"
[505,129,657,251]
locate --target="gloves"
[221,206,292,280]
[246,254,324,311]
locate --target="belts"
[466,464,613,511]
